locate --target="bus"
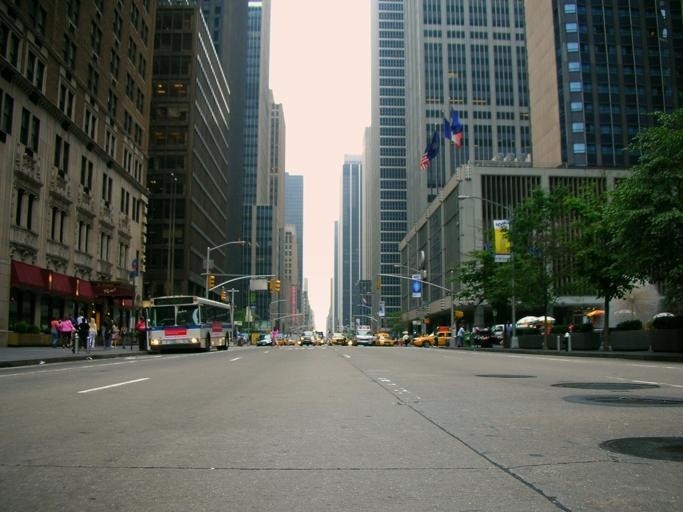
[144,294,234,355]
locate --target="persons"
[455,320,512,350]
[567,322,575,335]
[392,333,428,348]
[48,310,128,351]
[268,326,280,347]
[309,328,336,347]
[135,316,146,352]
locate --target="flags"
[442,119,461,148]
[449,109,463,141]
[418,142,431,171]
[421,129,440,165]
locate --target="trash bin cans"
[464,332,472,347]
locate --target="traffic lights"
[208,276,215,289]
[221,291,227,301]
[273,281,281,295]
[375,279,384,290]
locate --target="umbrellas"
[516,315,540,327]
[613,309,636,315]
[651,310,678,319]
[537,315,555,326]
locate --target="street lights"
[394,263,426,337]
[206,239,246,298]
[358,305,373,329]
[456,195,519,349]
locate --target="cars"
[412,327,452,347]
[491,322,509,345]
[256,329,395,347]
